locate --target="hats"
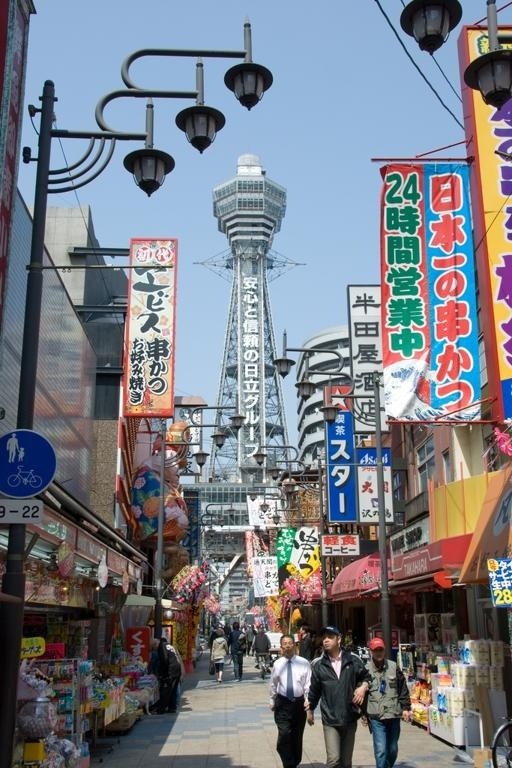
[319,625,339,637]
[368,637,386,651]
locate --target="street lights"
[154,392,246,655]
[0,15,300,763]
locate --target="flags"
[378,161,482,425]
[251,556,280,598]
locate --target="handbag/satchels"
[237,634,247,650]
[208,663,215,675]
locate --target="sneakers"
[157,709,176,714]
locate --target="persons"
[360,638,414,768]
[268,635,315,768]
[159,638,186,712]
[209,620,272,683]
[306,625,373,768]
[298,624,313,662]
[147,638,182,714]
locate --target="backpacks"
[168,654,182,678]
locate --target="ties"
[287,661,294,700]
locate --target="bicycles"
[490,716,512,768]
[257,655,270,680]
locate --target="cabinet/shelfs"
[428,691,509,747]
